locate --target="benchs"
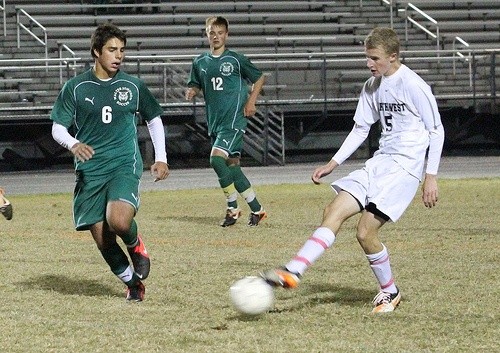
[0,0,500,113]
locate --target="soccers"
[228,275,277,320]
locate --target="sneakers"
[219,205,244,227]
[127,236,150,280]
[371,285,402,314]
[0,188,14,220]
[247,205,268,227]
[126,281,146,303]
[260,266,303,289]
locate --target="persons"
[257,26,445,313]
[185,16,268,227]
[50,25,169,303]
